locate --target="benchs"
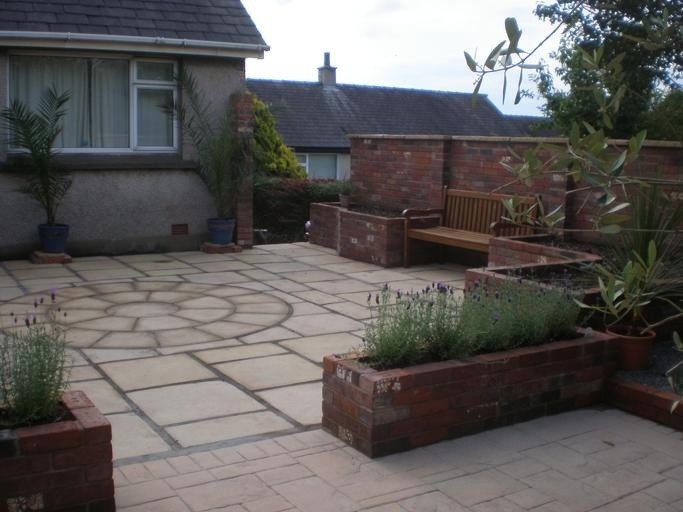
[403,186,542,268]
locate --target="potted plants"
[0,84,80,253]
[574,236,683,371]
[151,59,241,244]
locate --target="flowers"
[342,274,580,370]
[0,285,79,430]
